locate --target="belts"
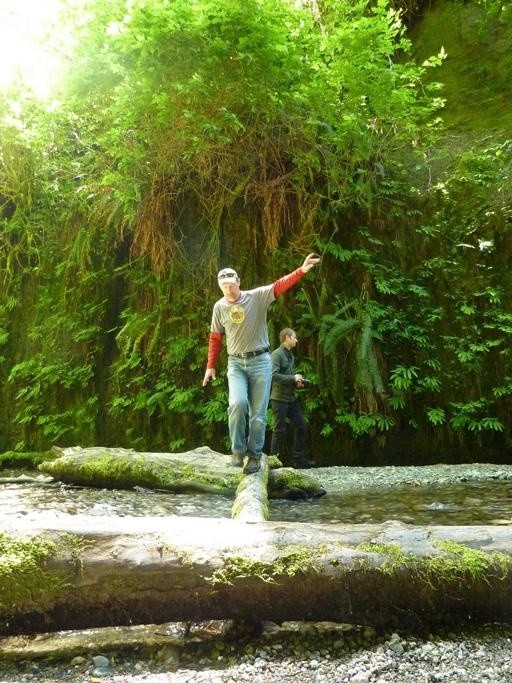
[229,348,269,358]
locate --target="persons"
[202,252,321,474]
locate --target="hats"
[218,268,238,286]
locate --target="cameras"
[302,379,310,389]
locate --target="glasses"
[219,273,234,279]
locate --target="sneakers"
[231,454,244,467]
[243,456,261,474]
[294,456,316,467]
